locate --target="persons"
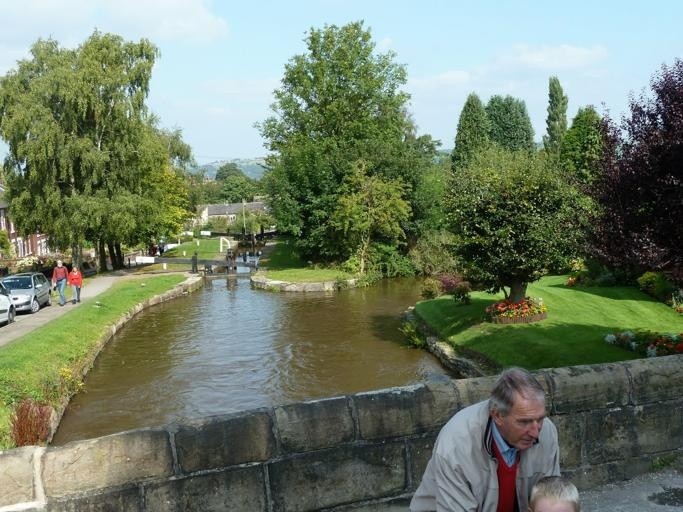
[526,474,584,512]
[50,258,70,306]
[64,265,84,303]
[190,250,199,276]
[147,234,167,256]
[406,365,562,512]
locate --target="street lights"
[242,199,246,235]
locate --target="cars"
[1,272,52,314]
[0,279,16,326]
